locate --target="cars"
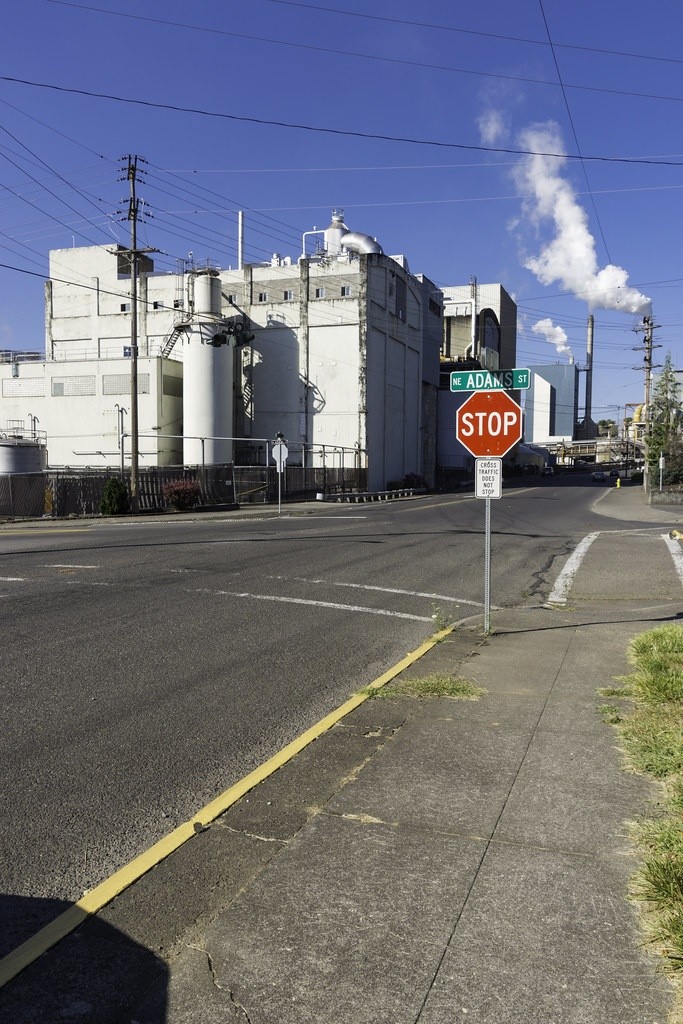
[610,470,619,477]
[593,472,606,482]
[540,466,554,476]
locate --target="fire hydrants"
[615,478,621,488]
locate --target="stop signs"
[456,390,523,459]
